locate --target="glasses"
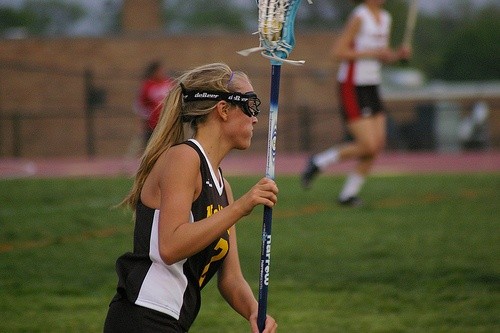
[220,88,262,117]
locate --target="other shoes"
[340,198,364,207]
[301,158,322,187]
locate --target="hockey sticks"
[251,0,308,333]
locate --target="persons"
[105,62,279,333]
[137,58,175,155]
[300,0,427,211]
